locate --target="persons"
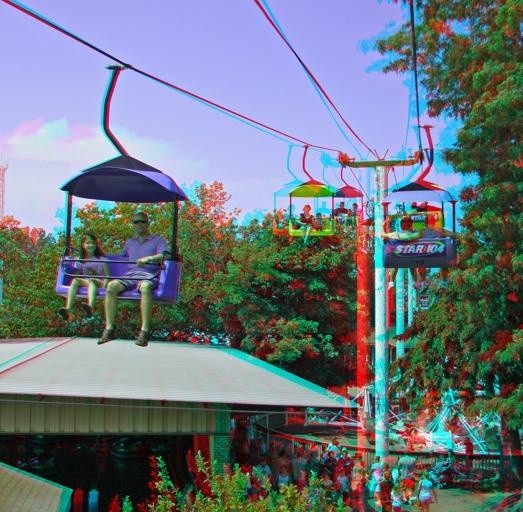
[73,389,522,510]
[94,213,167,347]
[57,230,105,321]
[291,198,429,247]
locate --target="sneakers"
[135,330,150,347]
[98,325,119,344]
[81,302,93,318]
[59,307,70,320]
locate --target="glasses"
[134,220,147,225]
